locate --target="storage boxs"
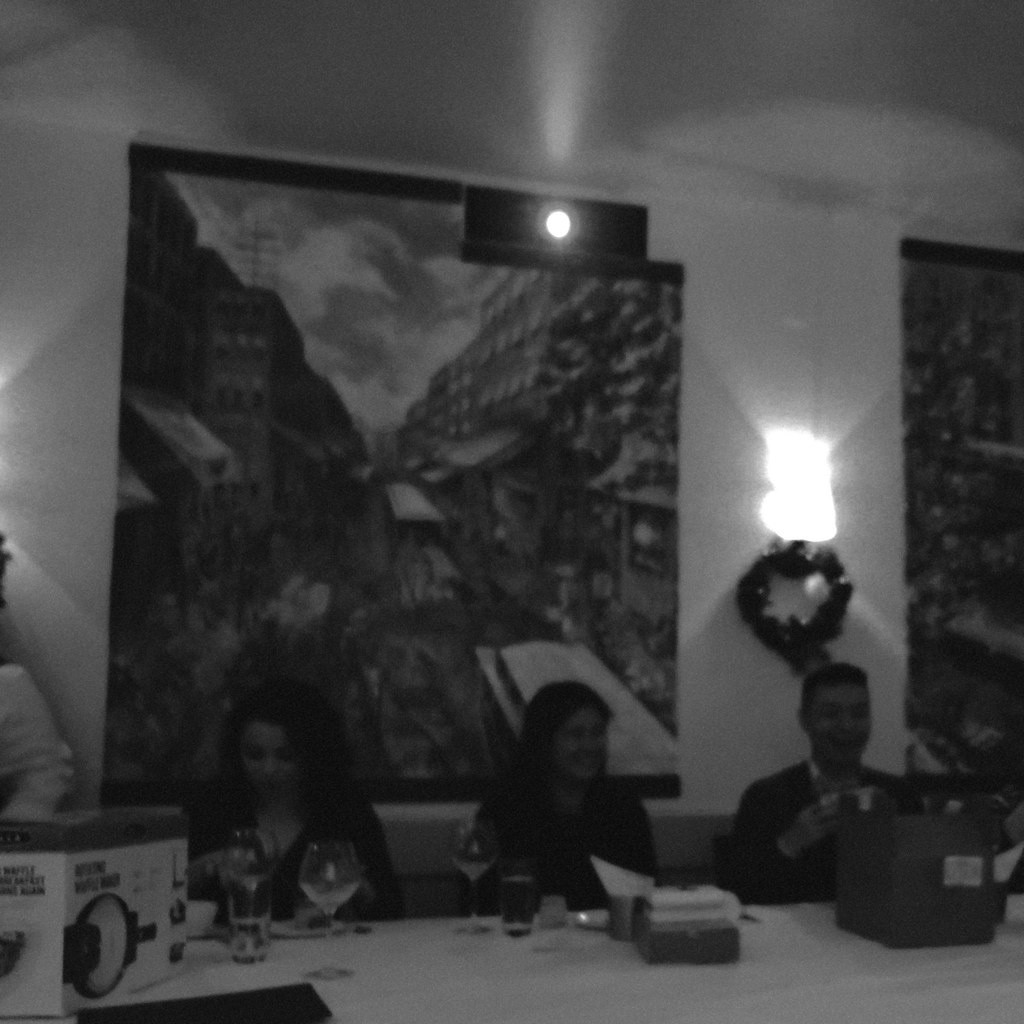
[832,813,1023,949]
[0,805,193,1017]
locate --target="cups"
[227,873,273,962]
[500,859,537,938]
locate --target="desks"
[0,896,1024,1023]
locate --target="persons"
[186,676,405,926]
[455,679,664,918]
[728,661,926,908]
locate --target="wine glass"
[448,819,498,936]
[299,839,361,980]
